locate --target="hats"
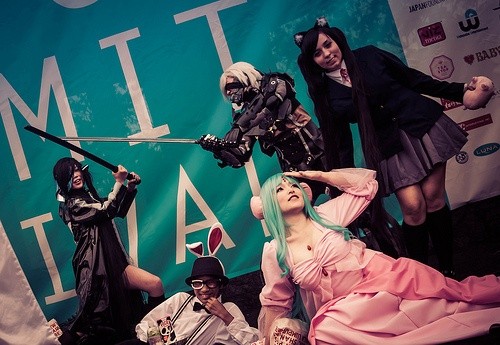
[185,257,229,287]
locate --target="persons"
[250,168,500,345]
[200,62,325,200]
[296,17,473,280]
[107,257,256,345]
[54,157,164,345]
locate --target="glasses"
[189,278,222,289]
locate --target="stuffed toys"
[463,75,500,111]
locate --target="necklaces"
[307,245,311,250]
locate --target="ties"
[340,68,351,84]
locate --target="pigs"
[461,75,497,111]
